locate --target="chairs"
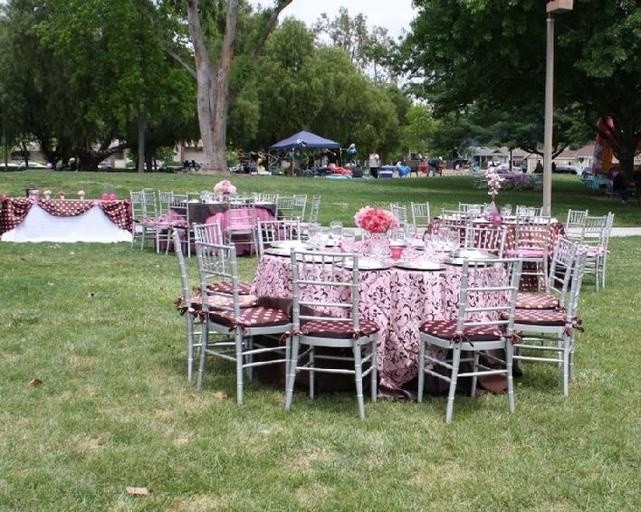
[392,200,615,295]
[417,253,524,423]
[284,247,380,421]
[191,235,292,409]
[257,217,300,249]
[171,228,251,381]
[376,161,444,178]
[129,187,321,259]
[502,237,578,367]
[191,220,258,348]
[500,243,588,396]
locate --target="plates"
[180,200,274,205]
[266,236,493,271]
[435,213,558,226]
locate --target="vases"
[43,195,85,199]
[366,234,394,260]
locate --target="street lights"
[543,0,574,219]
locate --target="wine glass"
[467,203,537,223]
[199,190,259,204]
[307,220,460,263]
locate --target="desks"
[257,243,509,402]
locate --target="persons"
[519,160,526,173]
[614,168,631,206]
[452,145,459,170]
[318,147,337,165]
[61,160,72,169]
[46,159,52,168]
[305,151,318,168]
[632,162,641,205]
[16,158,24,170]
[368,148,380,178]
[575,156,586,176]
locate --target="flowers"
[356,206,402,232]
[213,180,235,195]
[44,190,86,196]
